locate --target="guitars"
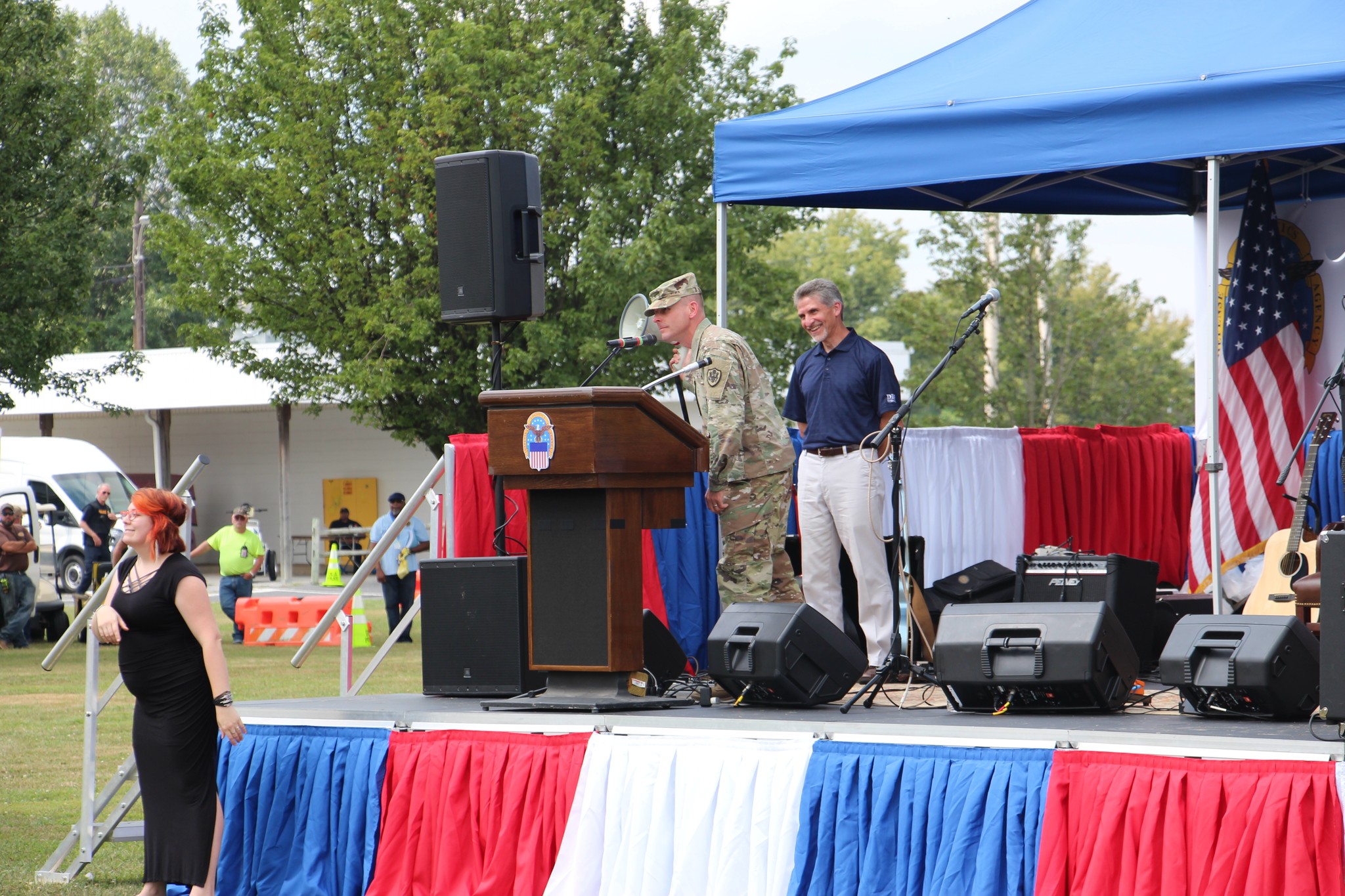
[1240,410,1340,618]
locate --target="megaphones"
[619,294,689,371]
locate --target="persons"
[641,269,808,697]
[90,488,248,895]
[369,492,430,643]
[77,482,117,593]
[12,506,42,535]
[189,506,265,646]
[782,277,904,682]
[0,503,37,650]
[327,507,366,574]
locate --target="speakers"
[433,150,545,324]
[642,608,687,697]
[933,529,1345,723]
[707,600,869,709]
[420,556,548,698]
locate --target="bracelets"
[212,690,234,707]
[249,571,256,579]
[22,539,27,546]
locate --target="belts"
[229,574,240,577]
[807,444,870,456]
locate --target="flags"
[1183,158,1314,600]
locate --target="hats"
[388,493,405,501]
[644,272,702,317]
[13,505,25,516]
[0,503,14,512]
[340,508,349,513]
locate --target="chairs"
[1292,521,1345,631]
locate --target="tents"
[706,0,1345,325]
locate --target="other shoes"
[0,640,7,649]
[691,684,735,703]
[232,634,243,644]
[859,665,894,683]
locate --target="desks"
[293,535,366,577]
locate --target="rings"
[235,726,240,730]
[232,731,236,735]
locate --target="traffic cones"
[350,588,376,649]
[319,543,345,588]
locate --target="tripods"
[840,306,987,714]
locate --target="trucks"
[0,436,139,594]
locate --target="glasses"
[0,512,14,516]
[102,492,110,495]
[119,510,143,521]
[390,500,403,503]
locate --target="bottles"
[353,524,358,536]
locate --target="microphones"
[606,333,657,348]
[641,357,712,391]
[960,288,1000,319]
